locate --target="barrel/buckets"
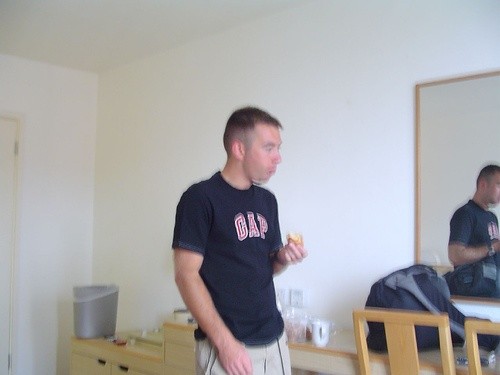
[72,285,119,339]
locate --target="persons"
[448,165,499,271]
[172,107,307,375]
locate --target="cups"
[313,321,329,347]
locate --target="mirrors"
[415,71,500,305]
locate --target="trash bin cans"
[72,285,119,339]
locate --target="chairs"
[353,308,457,375]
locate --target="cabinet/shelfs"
[70,328,165,375]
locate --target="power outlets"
[275,289,284,305]
[290,290,305,308]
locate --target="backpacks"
[442,261,500,299]
[366,264,500,354]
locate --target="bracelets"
[488,241,495,257]
[276,246,290,266]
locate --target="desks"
[289,325,500,375]
